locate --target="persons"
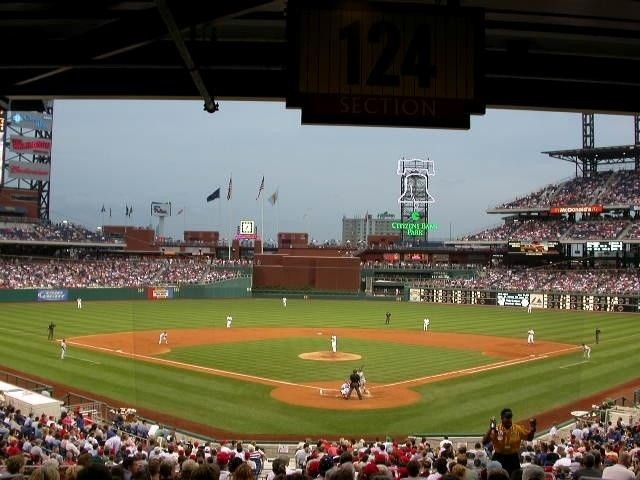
[582,344,591,360]
[520,402,640,480]
[341,368,367,400]
[269,453,308,479]
[68,408,185,479]
[385,312,391,324]
[158,331,169,345]
[295,436,508,480]
[60,340,66,359]
[0,220,254,290]
[282,296,287,307]
[77,296,82,309]
[527,328,534,344]
[528,303,532,314]
[331,335,337,352]
[596,328,600,343]
[0,388,85,480]
[158,440,267,479]
[360,170,640,301]
[48,321,56,341]
[482,408,537,479]
[226,314,232,328]
[423,317,429,331]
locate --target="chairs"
[0,406,106,458]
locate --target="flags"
[207,189,219,202]
[99,202,134,219]
[255,176,264,200]
[227,179,231,200]
[268,190,278,205]
